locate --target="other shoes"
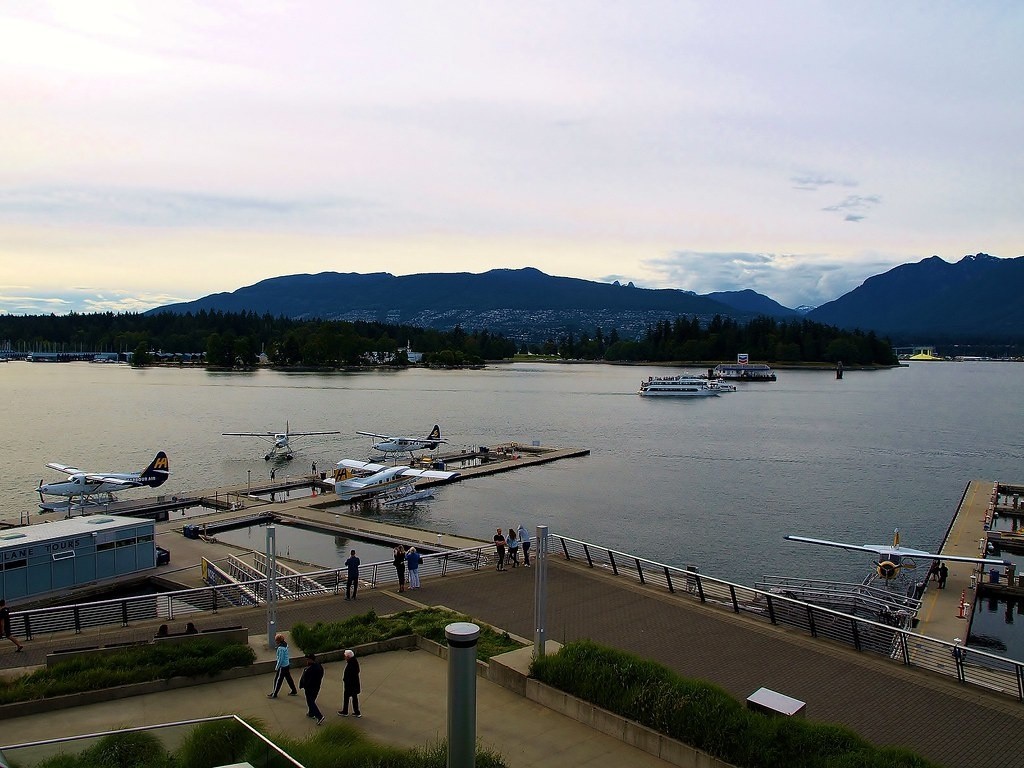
[351,596,355,599]
[305,712,315,719]
[288,692,297,696]
[352,712,361,718]
[407,585,420,590]
[337,710,349,717]
[266,693,276,698]
[495,567,507,571]
[343,596,350,600]
[525,564,530,568]
[316,716,325,725]
[396,589,404,592]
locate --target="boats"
[705,363,778,382]
[637,368,737,396]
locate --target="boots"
[512,563,515,568]
[516,561,520,568]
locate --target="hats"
[274,634,284,641]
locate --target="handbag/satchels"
[418,558,423,564]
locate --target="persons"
[931,560,941,580]
[298,653,325,725]
[344,549,360,600]
[393,544,406,592]
[269,468,278,482]
[516,524,531,567]
[183,623,198,635]
[154,625,169,638]
[505,528,520,568]
[0,599,23,652]
[405,547,420,589]
[312,461,318,475]
[494,528,508,571]
[266,634,298,699]
[936,563,949,588]
[337,650,362,718]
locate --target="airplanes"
[334,457,461,511]
[782,525,1012,603]
[222,420,341,461]
[355,423,450,466]
[34,450,173,511]
